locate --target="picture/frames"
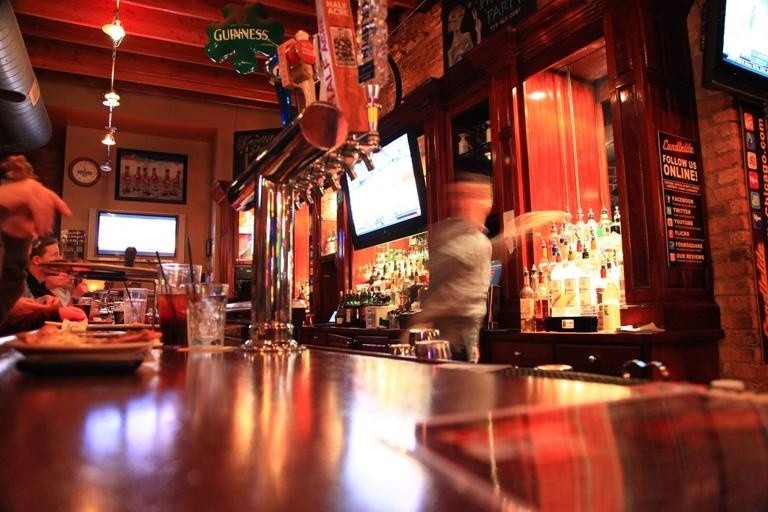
[114,148,188,205]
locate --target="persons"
[400,172,570,363]
[0,178,89,337]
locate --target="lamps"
[99,0,127,172]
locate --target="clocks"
[68,157,101,187]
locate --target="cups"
[123,287,147,325]
[184,282,226,347]
[71,297,92,321]
[156,263,202,344]
[113,302,124,323]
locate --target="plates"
[45,320,160,329]
[6,342,151,376]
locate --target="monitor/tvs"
[704,0,768,103]
[86,208,185,263]
[342,126,427,250]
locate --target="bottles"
[520,204,623,333]
[296,280,310,313]
[337,231,429,326]
[325,229,337,255]
